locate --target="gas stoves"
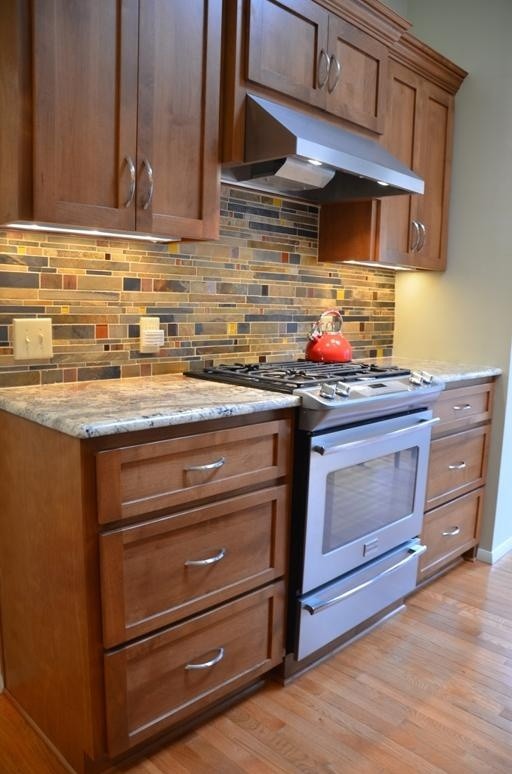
[183,357,445,435]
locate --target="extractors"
[218,93,427,204]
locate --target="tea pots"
[305,309,352,362]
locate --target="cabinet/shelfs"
[414,372,498,589]
[0,405,296,773]
[0,0,221,248]
[227,0,414,136]
[319,29,470,271]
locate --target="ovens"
[285,409,440,664]
